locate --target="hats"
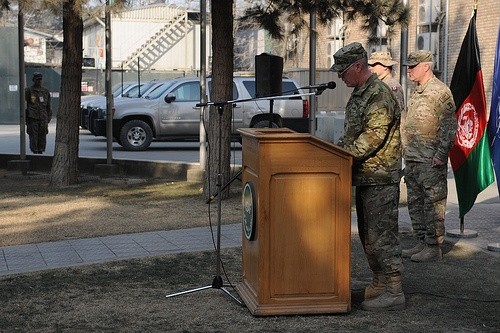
[330,42,367,72]
[34,73,43,79]
[403,49,433,67]
[367,51,398,66]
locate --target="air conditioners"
[324,10,344,38]
[366,16,388,37]
[368,45,388,59]
[416,32,440,70]
[417,0,441,24]
[325,40,343,68]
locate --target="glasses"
[338,60,359,78]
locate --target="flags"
[448,10,495,219]
[486,22,500,195]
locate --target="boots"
[352,272,387,301]
[362,274,406,312]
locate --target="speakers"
[255,53,284,98]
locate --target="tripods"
[165,90,325,307]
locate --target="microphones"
[299,81,337,89]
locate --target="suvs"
[80,76,317,151]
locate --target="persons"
[398,50,456,262]
[328,42,406,311]
[25,72,51,154]
[368,51,404,111]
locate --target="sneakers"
[401,242,426,258]
[411,244,443,262]
